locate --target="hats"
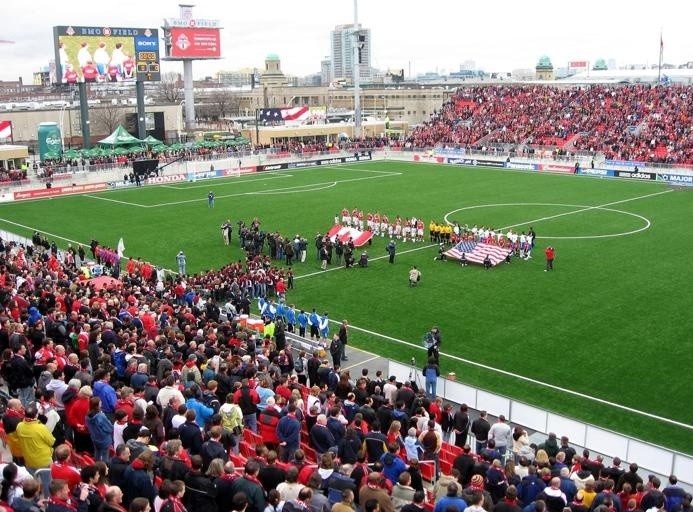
[471,474,483,486]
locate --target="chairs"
[410,86,693,168]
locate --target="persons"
[0,205,693,512]
[82,61,97,82]
[94,43,110,81]
[77,42,92,82]
[111,43,129,80]
[106,61,118,82]
[64,64,80,83]
[123,55,134,78]
[59,43,69,83]
[208,191,214,208]
[0,82,693,186]
[99,75,105,82]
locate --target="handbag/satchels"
[417,276,421,281]
[233,426,242,436]
